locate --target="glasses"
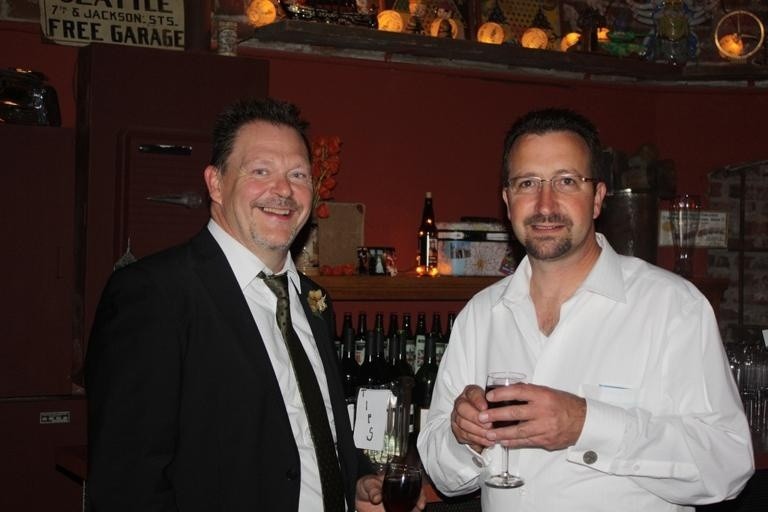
[507,172,597,195]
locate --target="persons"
[82,97,427,512]
[416,107,757,511]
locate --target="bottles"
[725,338,768,436]
[416,190,438,274]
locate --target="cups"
[334,309,457,438]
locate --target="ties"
[259,270,347,511]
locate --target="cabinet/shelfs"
[0,39,271,512]
[183,0,768,297]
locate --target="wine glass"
[484,371,527,489]
[381,464,422,512]
